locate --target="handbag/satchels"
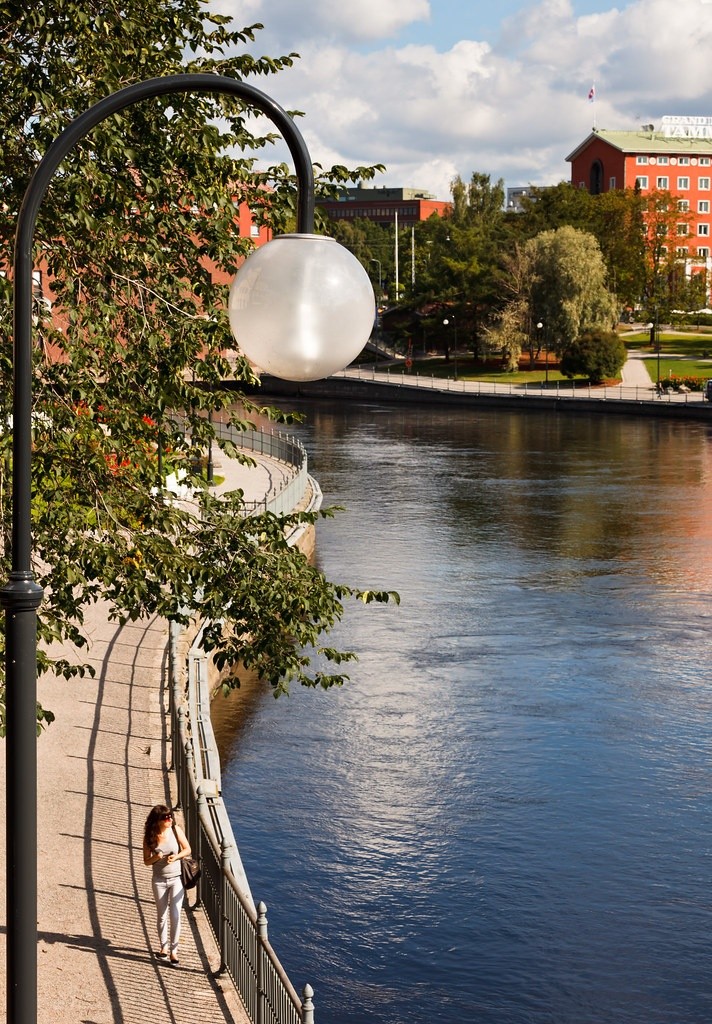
[171,823,200,890]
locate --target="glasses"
[159,814,172,820]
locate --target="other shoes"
[170,955,179,964]
[159,953,168,960]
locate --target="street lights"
[2,73,377,1024]
[370,258,381,308]
[538,317,550,387]
[647,309,661,394]
[443,314,459,381]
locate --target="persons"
[656,385,664,400]
[143,804,192,964]
